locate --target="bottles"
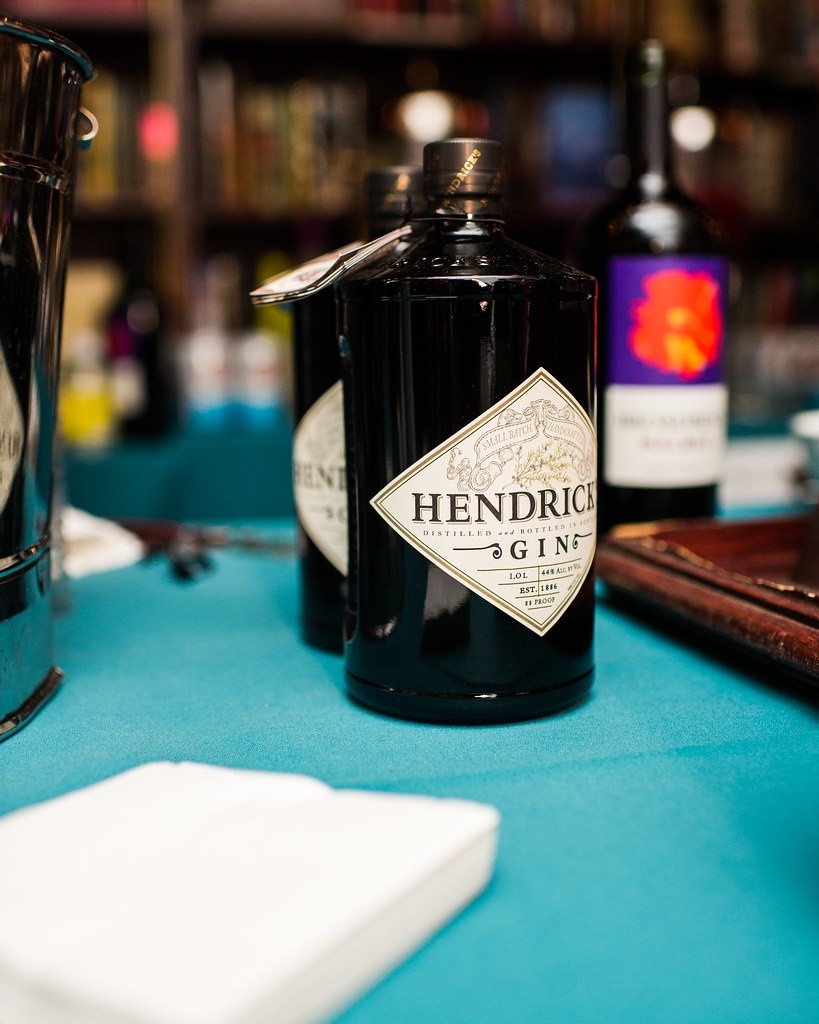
[292,167,426,657]
[596,39,729,534]
[339,140,596,725]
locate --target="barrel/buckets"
[0,18,97,720]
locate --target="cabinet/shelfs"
[0,0,819,453]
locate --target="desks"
[0,501,819,1024]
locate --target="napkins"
[0,761,500,1024]
